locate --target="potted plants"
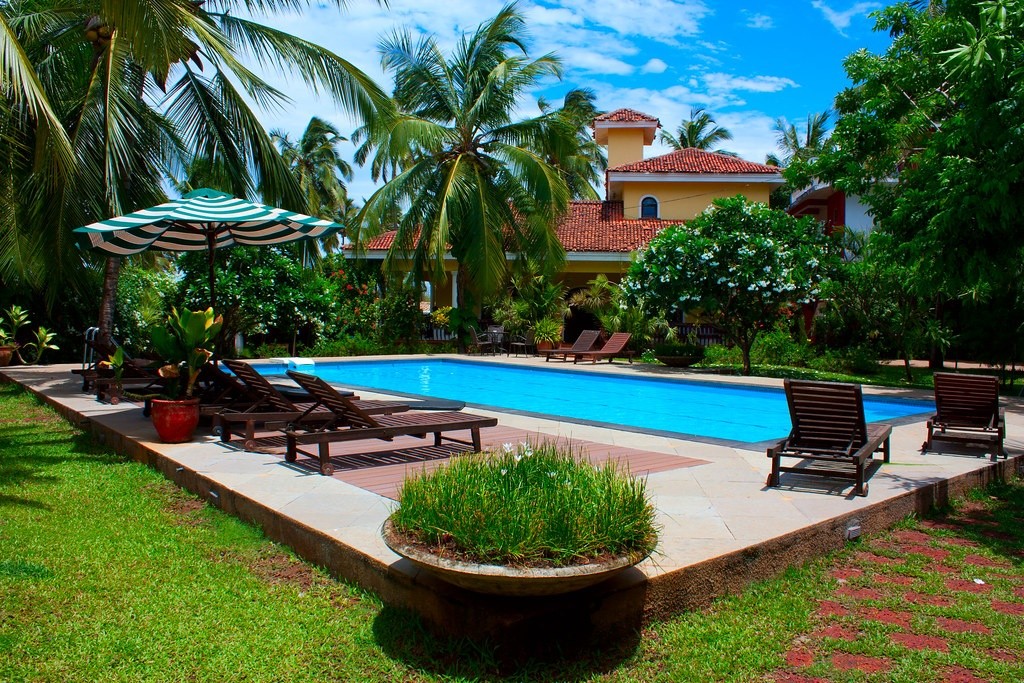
[655,340,707,367]
[153,306,222,444]
[0,303,60,366]
[533,320,563,355]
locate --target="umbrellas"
[73,187,348,367]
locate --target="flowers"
[381,434,657,569]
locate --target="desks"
[481,331,509,356]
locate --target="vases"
[381,510,659,597]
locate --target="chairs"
[284,370,497,474]
[509,329,535,358]
[468,325,491,356]
[216,358,409,452]
[71,356,155,403]
[487,324,504,355]
[925,372,1008,461]
[766,378,893,499]
[202,361,360,433]
[539,331,601,363]
[571,332,636,364]
